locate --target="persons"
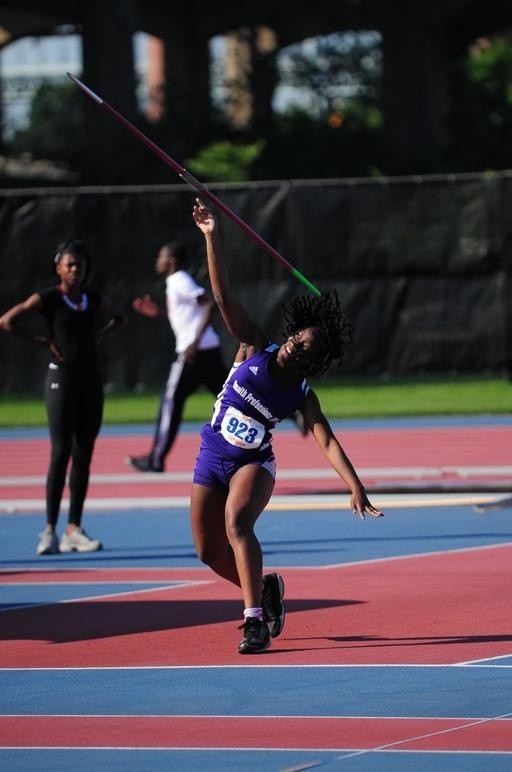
[124,240,310,473]
[187,197,386,653]
[0,239,130,555]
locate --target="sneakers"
[126,456,149,471]
[237,617,271,654]
[261,573,285,638]
[37,529,59,555]
[58,528,102,552]
